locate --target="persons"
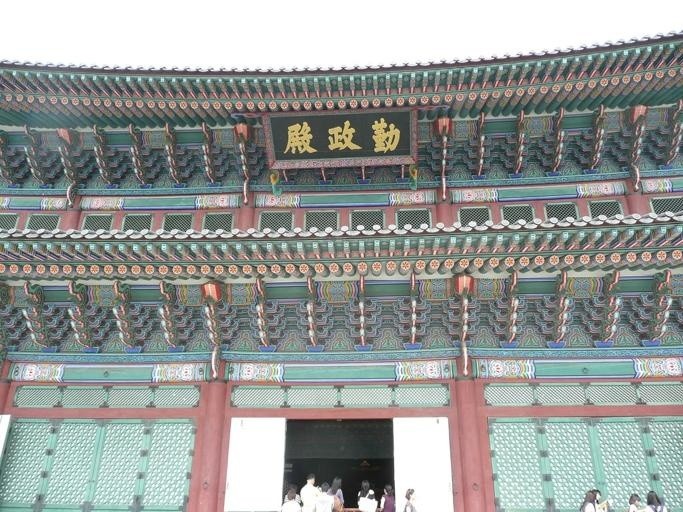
[629,491,667,512]
[581,489,607,512]
[357,480,394,512]
[281,472,345,512]
[404,488,417,512]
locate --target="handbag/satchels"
[358,493,378,512]
[334,496,343,512]
